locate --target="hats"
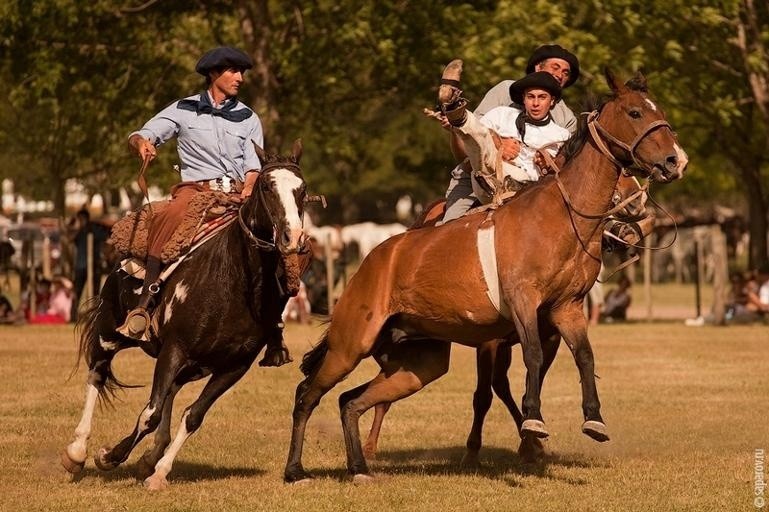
[526,45,579,88]
[196,48,251,76]
[509,71,561,105]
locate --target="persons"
[723,270,748,305]
[28,278,51,313]
[67,208,112,323]
[26,276,73,324]
[601,275,632,321]
[437,58,573,206]
[424,44,580,227]
[125,47,293,366]
[745,265,769,325]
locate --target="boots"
[128,314,147,334]
[438,60,463,106]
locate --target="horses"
[58,136,312,492]
[281,61,691,485]
